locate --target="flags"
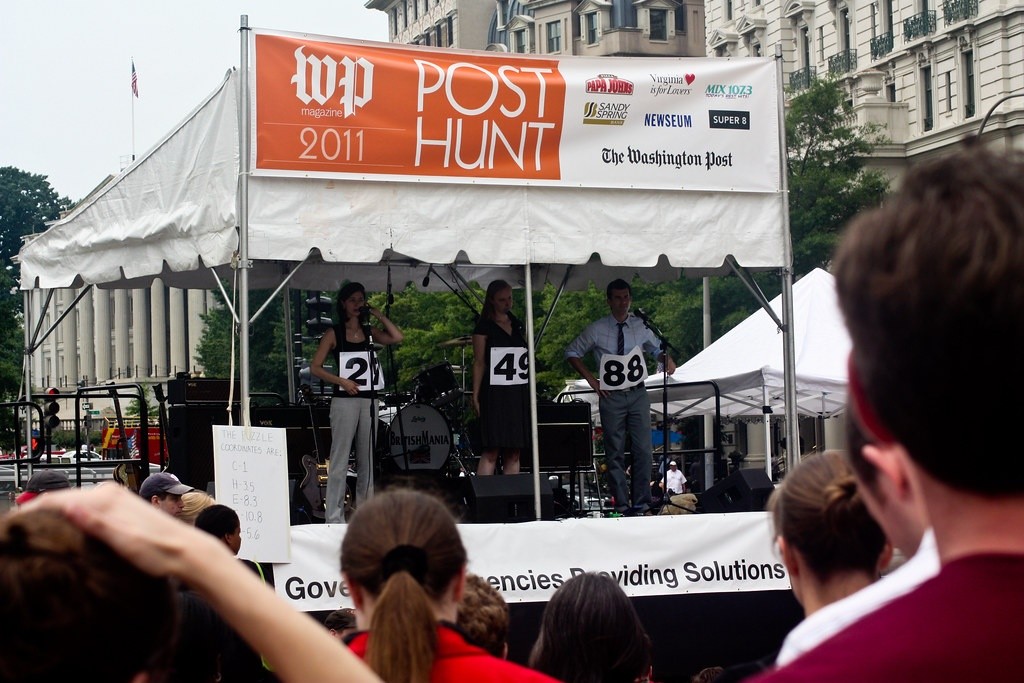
[132,63,138,97]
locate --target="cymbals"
[436,333,477,347]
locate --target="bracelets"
[379,314,383,319]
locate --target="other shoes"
[637,510,652,516]
[621,509,633,517]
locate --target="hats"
[139,472,195,500]
[16,468,70,505]
[670,461,676,465]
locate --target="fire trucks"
[100,416,169,465]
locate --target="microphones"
[388,268,394,304]
[506,310,525,330]
[359,306,372,315]
[633,309,651,322]
[422,264,433,287]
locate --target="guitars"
[294,382,353,521]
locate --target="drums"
[378,401,456,473]
[413,361,463,408]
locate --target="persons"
[8,454,12,460]
[769,447,894,617]
[566,278,675,516]
[650,473,664,497]
[662,460,687,494]
[55,445,66,452]
[0,479,733,683]
[745,145,1024,683]
[140,473,195,516]
[472,280,527,476]
[775,402,940,666]
[22,451,27,459]
[310,282,403,524]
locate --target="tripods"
[641,321,700,516]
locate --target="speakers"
[454,475,553,523]
[519,400,594,471]
[692,467,776,513]
[167,404,240,491]
[251,406,334,474]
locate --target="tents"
[18,16,797,479]
[558,268,853,481]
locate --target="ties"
[616,322,625,355]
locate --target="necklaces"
[352,332,359,338]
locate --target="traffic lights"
[44,387,61,429]
[30,437,41,454]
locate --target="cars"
[0,445,109,464]
